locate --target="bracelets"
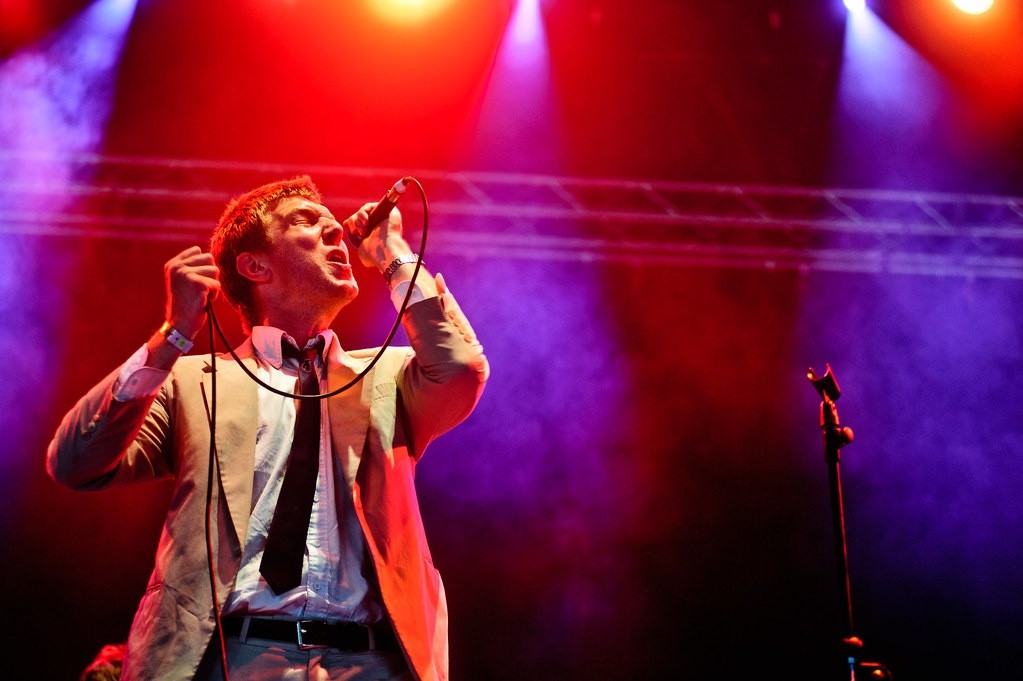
[160,322,194,354]
[383,254,426,285]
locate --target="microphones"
[346,177,408,247]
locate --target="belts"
[221,615,398,652]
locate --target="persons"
[80,643,127,681]
[45,176,491,681]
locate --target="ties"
[259,340,324,595]
